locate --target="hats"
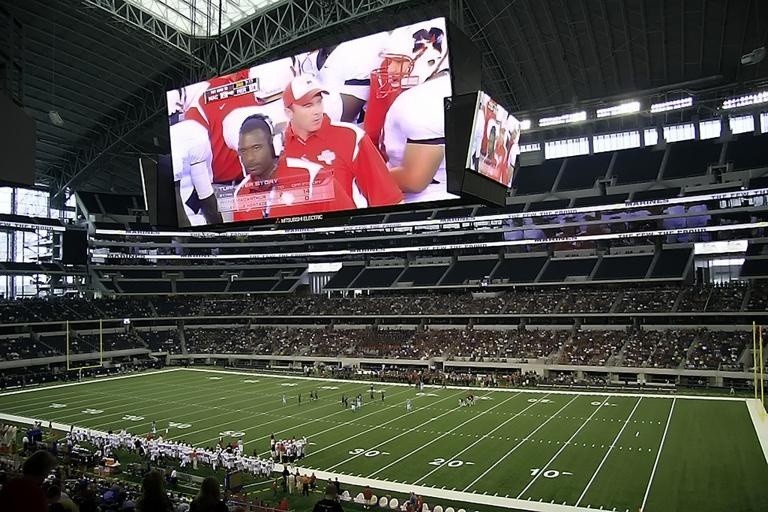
[283,74,329,107]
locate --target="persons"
[166,16,454,227]
[0,421,424,511]
[456,394,476,407]
[282,393,287,406]
[298,392,302,405]
[314,390,319,400]
[340,389,424,413]
[1,276,767,389]
[308,391,313,402]
[467,92,520,188]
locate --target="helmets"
[248,57,298,100]
[167,88,186,116]
[506,116,520,144]
[371,18,447,99]
